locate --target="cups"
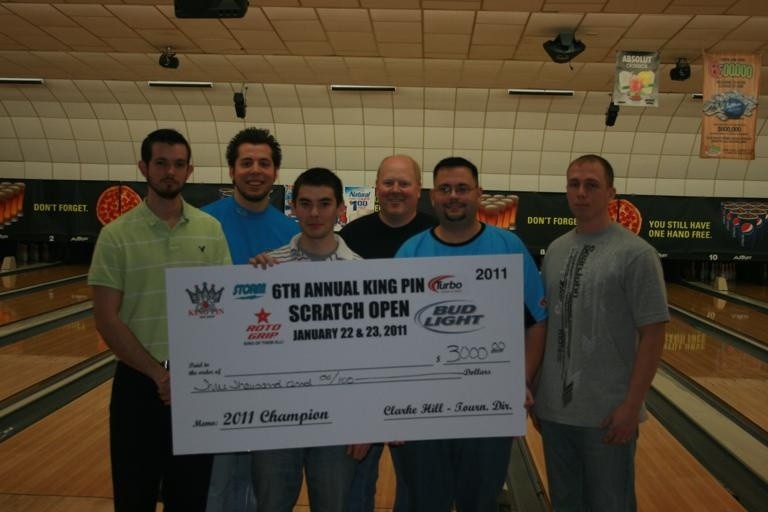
[477,193,521,231]
[0,181,26,231]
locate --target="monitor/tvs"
[174,0,247,18]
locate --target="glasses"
[432,184,475,195]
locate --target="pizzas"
[95,183,141,226]
[608,198,643,235]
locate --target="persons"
[531,155,669,511]
[195,127,301,511]
[251,164,374,511]
[339,153,441,509]
[389,157,548,509]
[86,127,235,511]
